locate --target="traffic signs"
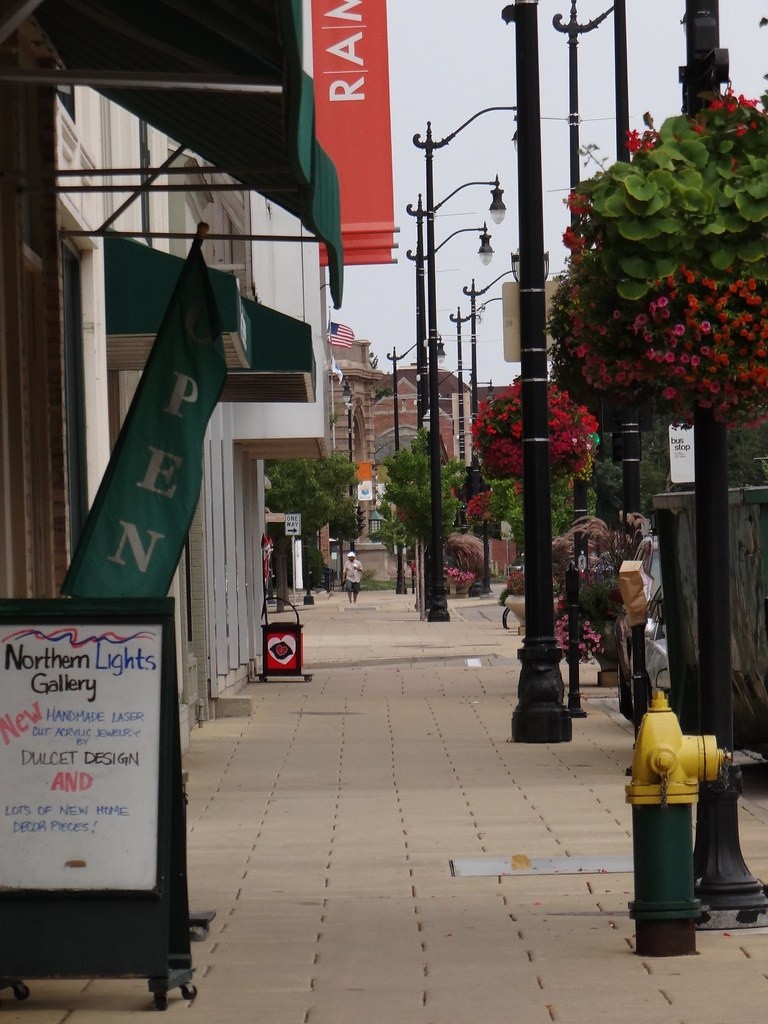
[284,512,303,537]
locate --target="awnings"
[220,295,316,402]
[0,1,314,205]
[102,227,251,372]
[60,69,344,310]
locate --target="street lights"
[340,379,359,597]
[402,103,553,626]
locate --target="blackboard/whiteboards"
[0,593,191,978]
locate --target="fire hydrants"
[614,688,733,956]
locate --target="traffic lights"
[355,505,368,536]
[570,386,606,466]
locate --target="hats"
[346,552,355,558]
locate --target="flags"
[62,245,227,596]
[331,357,344,384]
[326,321,356,349]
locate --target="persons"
[342,552,362,604]
